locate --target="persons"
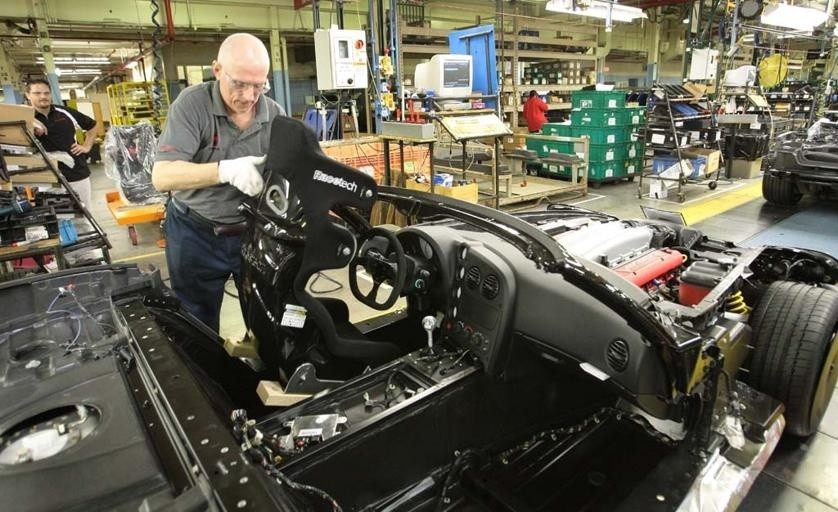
[522,91,549,133]
[25,75,99,221]
[150,32,288,336]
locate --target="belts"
[171,195,218,227]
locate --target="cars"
[760,110,837,205]
[0,114,837,512]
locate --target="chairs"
[241,119,399,394]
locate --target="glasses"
[220,66,271,95]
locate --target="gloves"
[218,154,266,196]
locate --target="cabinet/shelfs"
[638,85,726,204]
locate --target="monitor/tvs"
[414,54,473,97]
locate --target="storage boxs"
[652,148,720,178]
[498,60,646,180]
